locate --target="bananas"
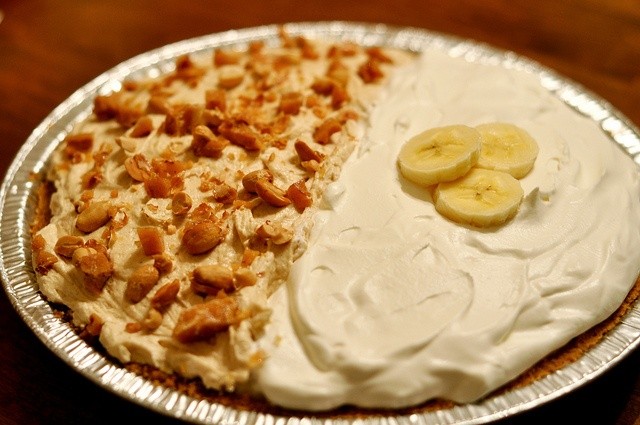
[433,166,525,228]
[397,124,481,187]
[474,122,539,179]
